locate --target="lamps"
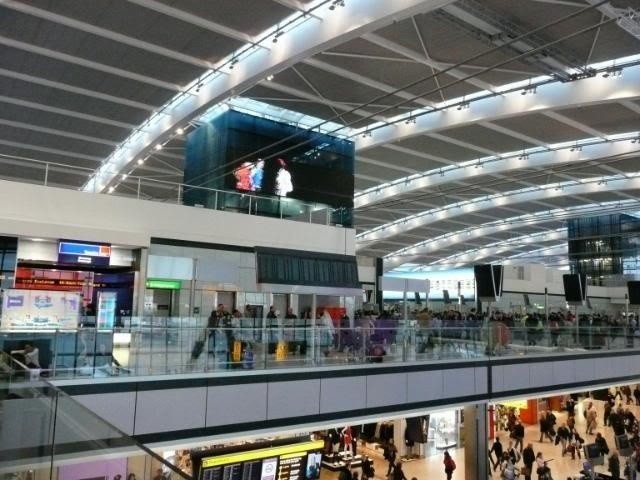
[79,0,640,260]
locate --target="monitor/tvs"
[474,264,497,302]
[563,274,583,305]
[580,274,587,301]
[627,281,640,304]
[492,265,504,298]
[0,235,19,254]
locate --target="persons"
[233,162,254,209]
[249,158,266,211]
[13,341,42,378]
[208,300,640,370]
[126,472,137,480]
[270,158,294,214]
[77,303,96,360]
[113,474,123,479]
[152,468,166,480]
[326,384,640,480]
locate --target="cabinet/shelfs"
[325,450,369,464]
